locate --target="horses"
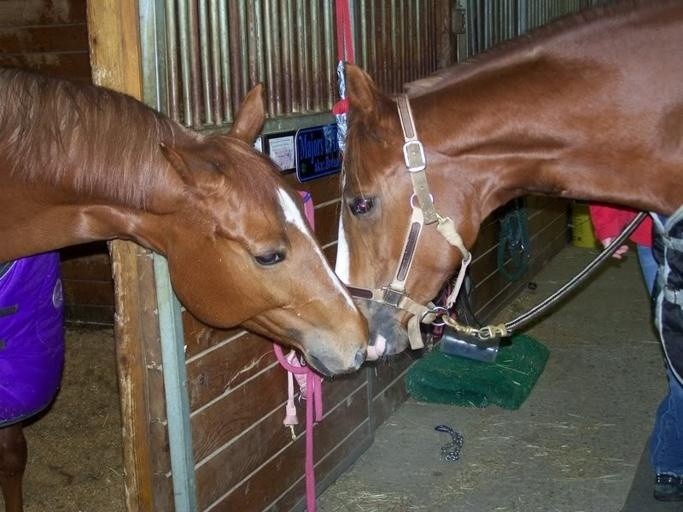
[0,61,371,511]
[334,0,683,363]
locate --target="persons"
[588,205,682,503]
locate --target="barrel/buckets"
[566,202,603,249]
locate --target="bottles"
[438,324,501,364]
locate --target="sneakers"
[654,474,682,501]
